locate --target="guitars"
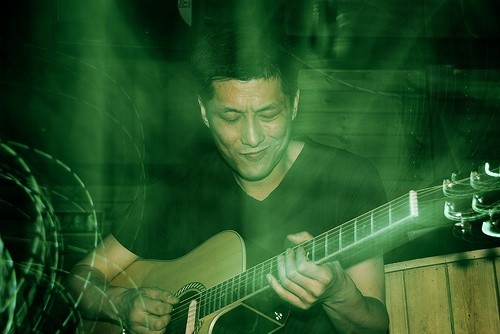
[76,163,500,334]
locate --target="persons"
[66,16,390,334]
